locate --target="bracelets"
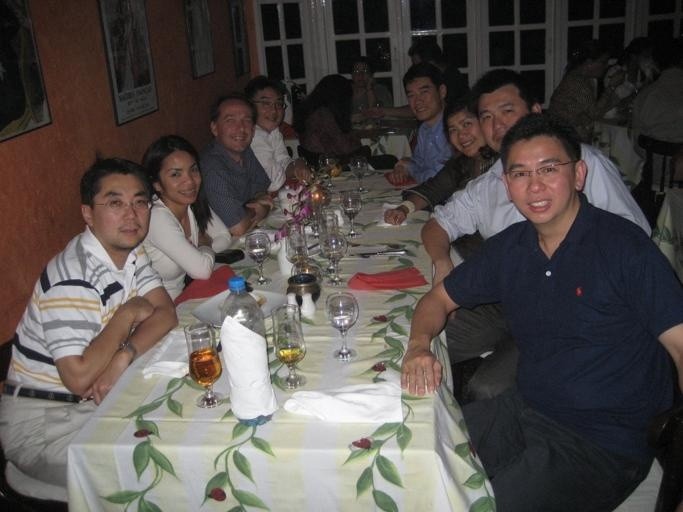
[364,87,374,94]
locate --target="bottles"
[221,279,274,425]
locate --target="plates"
[189,286,288,330]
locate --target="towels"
[142,324,220,380]
[375,202,408,227]
[282,380,404,424]
[174,265,237,306]
[347,266,429,291]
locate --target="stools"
[634,132,680,201]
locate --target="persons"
[382,93,498,225]
[1,155,179,490]
[602,37,659,120]
[393,61,451,191]
[549,39,633,146]
[365,38,476,120]
[419,67,653,289]
[197,92,274,240]
[293,73,399,171]
[402,110,683,512]
[629,38,682,230]
[139,133,235,304]
[349,52,394,125]
[242,74,313,197]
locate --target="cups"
[284,223,308,284]
[319,231,349,288]
[341,191,361,237]
[319,154,335,189]
[324,291,359,363]
[321,214,339,252]
[184,324,216,356]
[352,156,368,195]
[188,345,223,409]
[271,303,307,392]
[244,232,272,285]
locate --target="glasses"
[251,100,289,111]
[505,159,579,180]
[89,194,154,214]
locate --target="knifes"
[349,248,408,257]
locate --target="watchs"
[122,338,137,361]
